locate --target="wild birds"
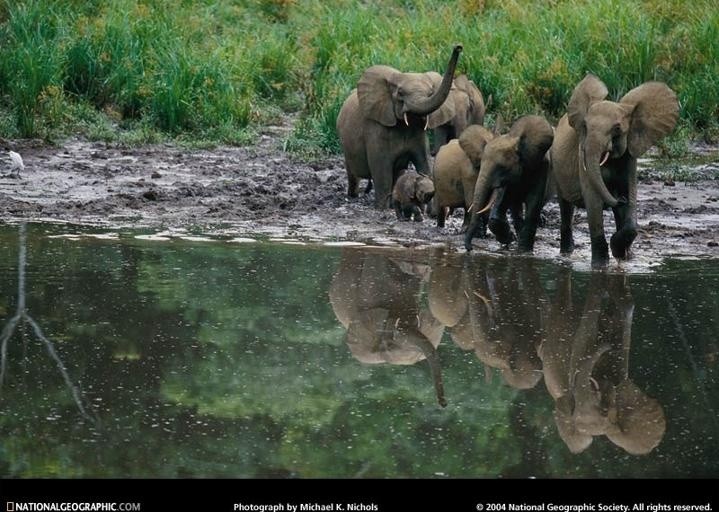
[4,150,25,177]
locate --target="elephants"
[335,44,682,272]
[325,244,665,457]
